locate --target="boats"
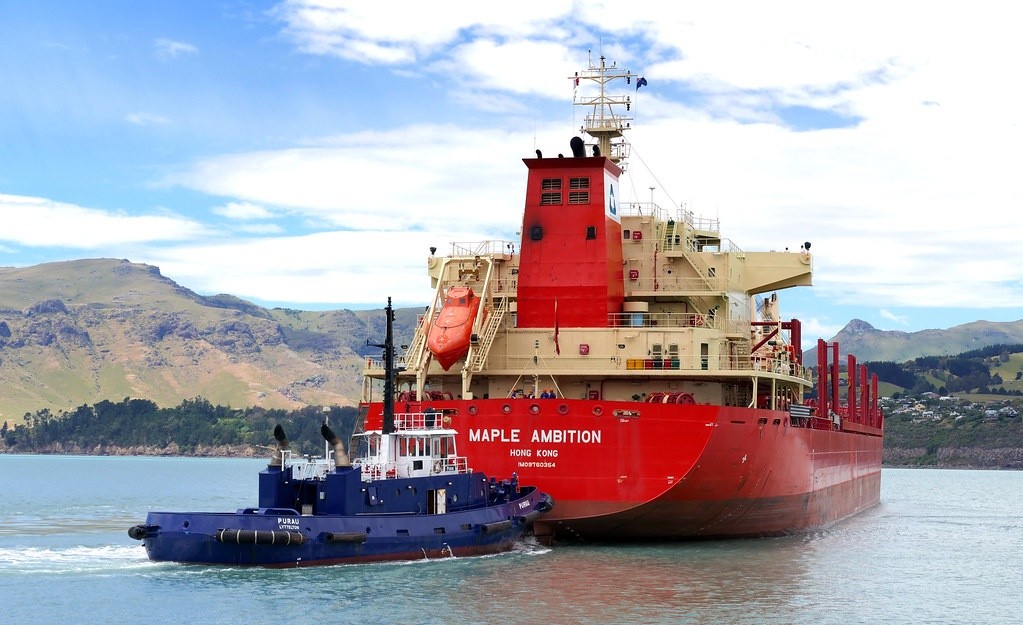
[429,288,487,371]
[129,297,555,568]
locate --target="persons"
[541,388,549,398]
[550,388,556,398]
[512,388,516,399]
[800,245,806,253]
[530,390,533,395]
[784,248,789,252]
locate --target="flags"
[637,77,647,88]
[555,301,560,354]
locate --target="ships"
[348,37,884,543]
